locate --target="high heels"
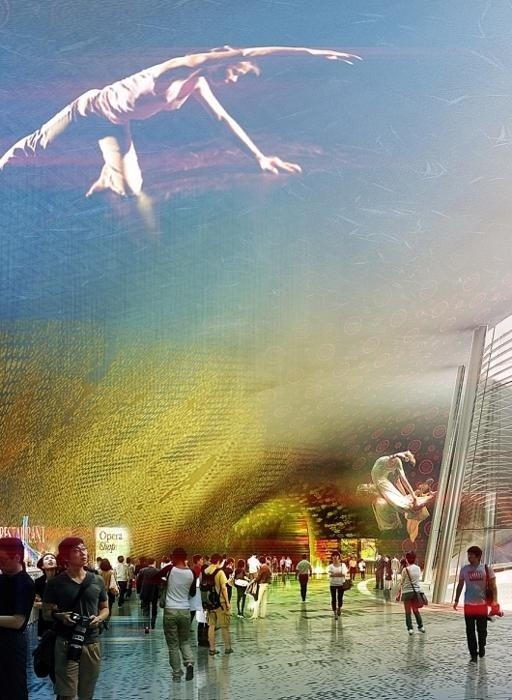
[240,615,245,619]
[237,614,240,617]
[337,609,341,616]
[335,613,338,620]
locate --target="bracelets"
[455,600,458,603]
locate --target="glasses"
[70,547,87,553]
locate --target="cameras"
[67,614,92,661]
[489,604,504,619]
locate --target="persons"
[327,552,349,619]
[453,546,499,666]
[348,556,366,581]
[0,536,292,700]
[295,554,312,601]
[400,550,425,634]
[356,450,438,543]
[374,553,407,589]
[1,44,363,200]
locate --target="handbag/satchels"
[109,573,120,596]
[235,579,249,588]
[486,588,495,606]
[32,630,58,678]
[342,579,353,591]
[227,577,234,587]
[415,592,429,607]
[157,582,167,608]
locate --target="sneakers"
[247,616,258,620]
[185,663,194,681]
[469,656,478,665]
[197,640,210,648]
[478,646,486,657]
[419,626,426,634]
[172,676,182,683]
[408,629,414,635]
[118,606,123,609]
[144,627,149,634]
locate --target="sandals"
[224,647,234,655]
[208,648,220,656]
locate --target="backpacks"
[200,574,222,610]
